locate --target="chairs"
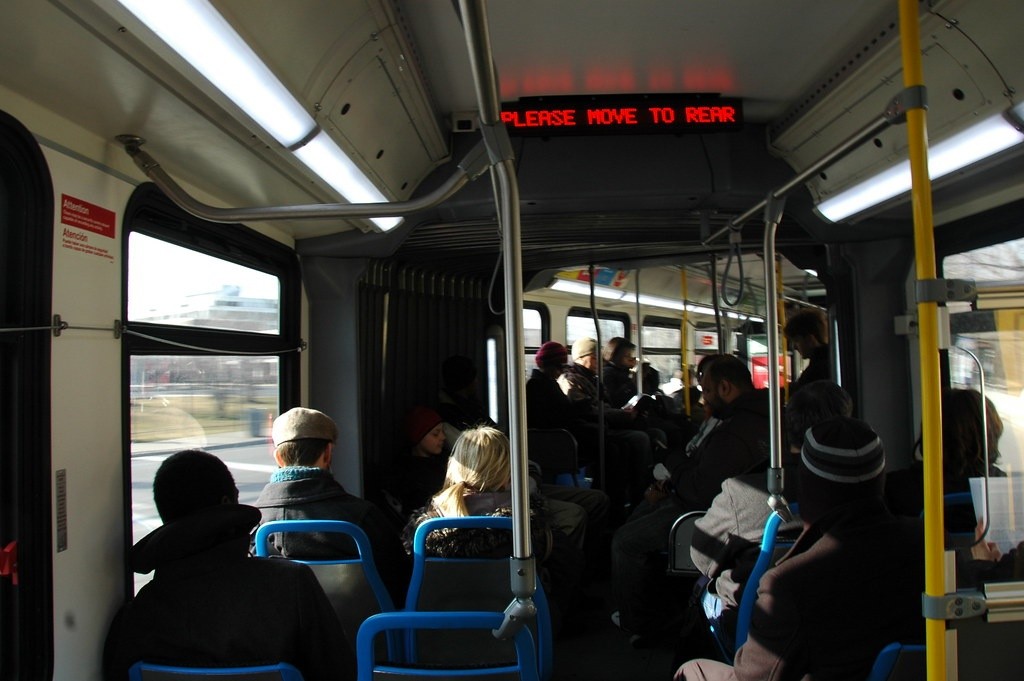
[128,492,974,681]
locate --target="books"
[967,476,1024,557]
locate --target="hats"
[273,407,338,445]
[402,406,443,450]
[798,418,886,507]
[571,337,596,360]
[536,341,567,366]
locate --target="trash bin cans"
[246,408,263,438]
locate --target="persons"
[784,307,830,386]
[99,450,359,681]
[609,352,1024,681]
[248,407,610,610]
[525,335,700,531]
